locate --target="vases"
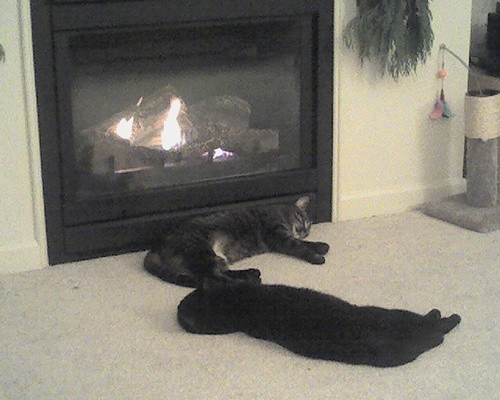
[464,90,500,208]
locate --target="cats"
[177,283,462,368]
[143,194,330,289]
